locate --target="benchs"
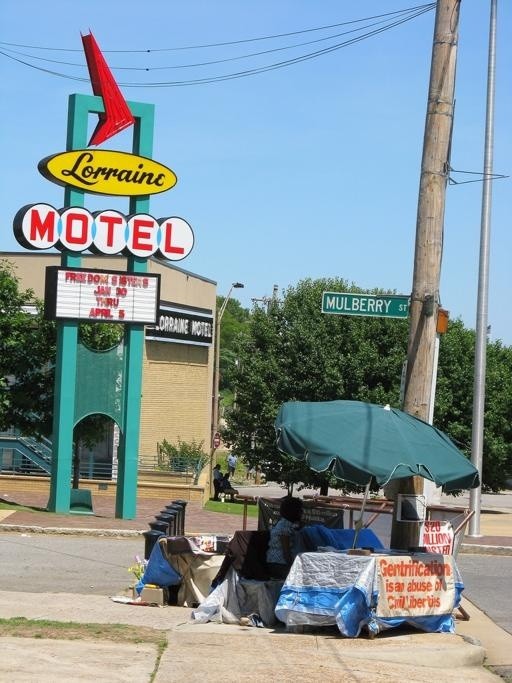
[207,528,384,629]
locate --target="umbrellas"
[272,399,483,548]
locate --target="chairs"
[219,489,231,499]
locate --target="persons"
[222,473,238,502]
[226,451,238,480]
[265,496,306,579]
[213,463,221,500]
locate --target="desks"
[274,549,465,638]
